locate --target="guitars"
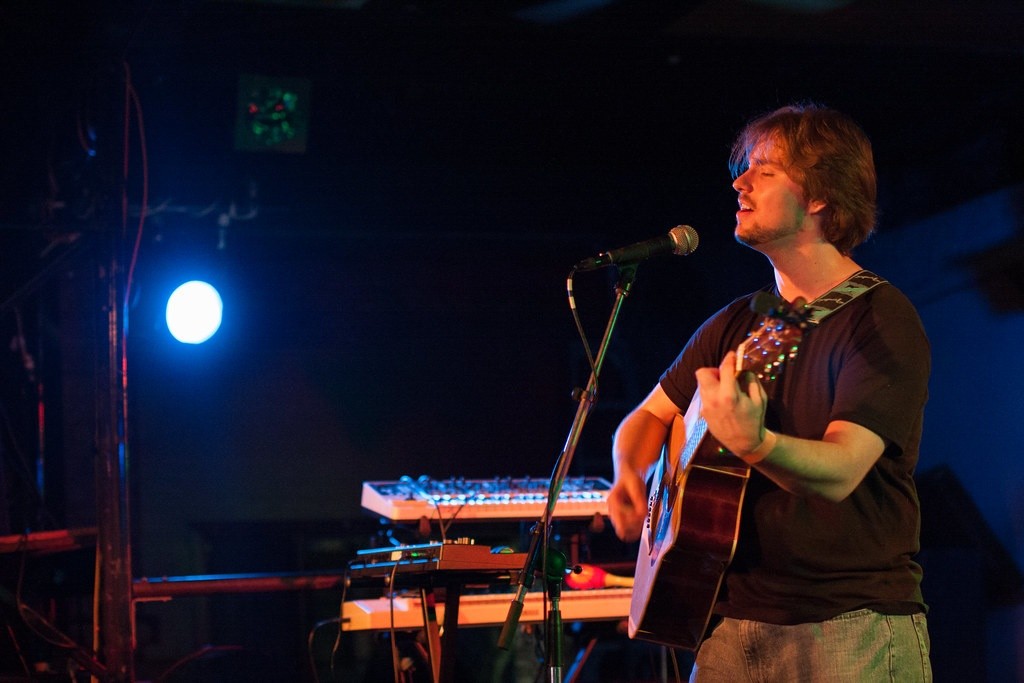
[626,296,803,649]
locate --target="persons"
[606,104,932,683]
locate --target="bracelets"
[739,428,776,465]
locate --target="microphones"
[574,226,699,272]
